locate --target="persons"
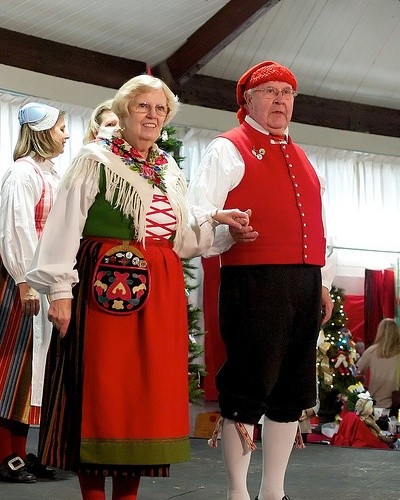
[0,99,67,483]
[29,70,249,500]
[186,59,335,500]
[81,98,124,141]
[352,317,400,417]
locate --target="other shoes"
[0,454,57,484]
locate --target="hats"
[17,103,61,131]
[236,60,297,124]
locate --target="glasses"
[128,102,169,117]
[249,86,299,100]
